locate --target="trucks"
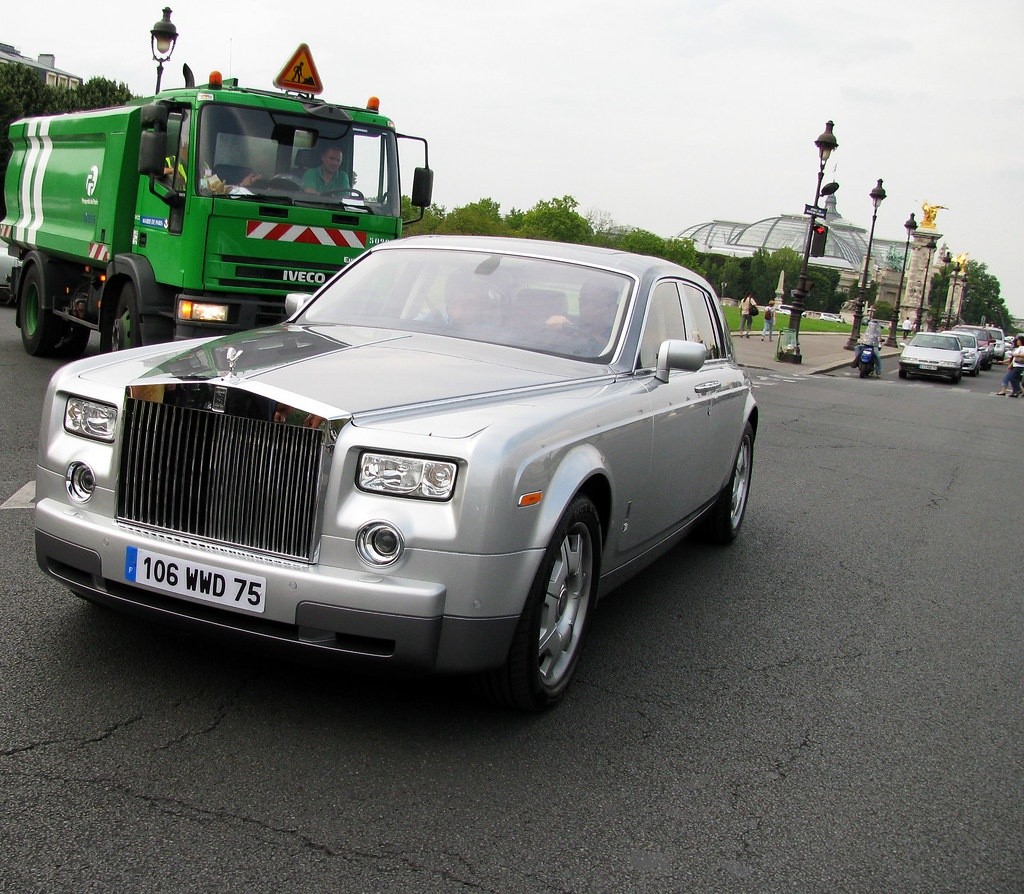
[1,62,433,358]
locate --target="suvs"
[952,324,1017,370]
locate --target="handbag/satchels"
[1015,349,1024,364]
[749,298,758,316]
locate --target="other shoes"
[740,334,743,338]
[996,393,1005,396]
[877,373,881,377]
[762,338,764,341]
[745,333,749,338]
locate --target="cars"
[36,234,759,719]
[898,332,969,385]
[0,238,24,305]
[776,305,844,323]
[938,331,985,377]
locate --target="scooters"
[857,337,885,378]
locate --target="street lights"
[883,211,919,348]
[933,250,967,333]
[843,178,888,350]
[778,119,839,363]
[150,5,179,94]
[914,236,937,334]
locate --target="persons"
[302,144,350,197]
[739,291,758,338]
[761,300,776,342]
[902,317,911,340]
[855,320,882,377]
[163,140,261,187]
[546,276,618,347]
[996,336,1024,398]
[415,271,470,324]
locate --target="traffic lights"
[811,222,828,258]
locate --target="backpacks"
[765,308,773,320]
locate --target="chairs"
[943,339,955,350]
[516,287,569,323]
[290,149,324,180]
[963,338,974,348]
[212,164,253,186]
[977,331,987,340]
